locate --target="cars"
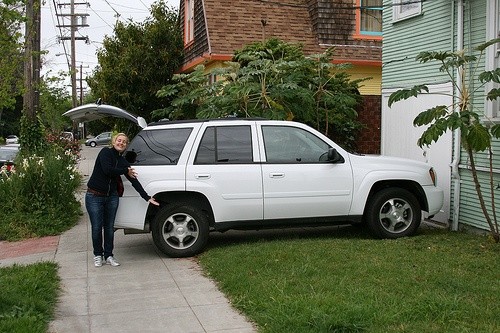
[85,132,114,147]
[5,135,19,145]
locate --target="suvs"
[61,98,445,257]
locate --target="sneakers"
[106,255,120,267]
[93,256,103,267]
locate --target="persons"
[85,133,160,267]
